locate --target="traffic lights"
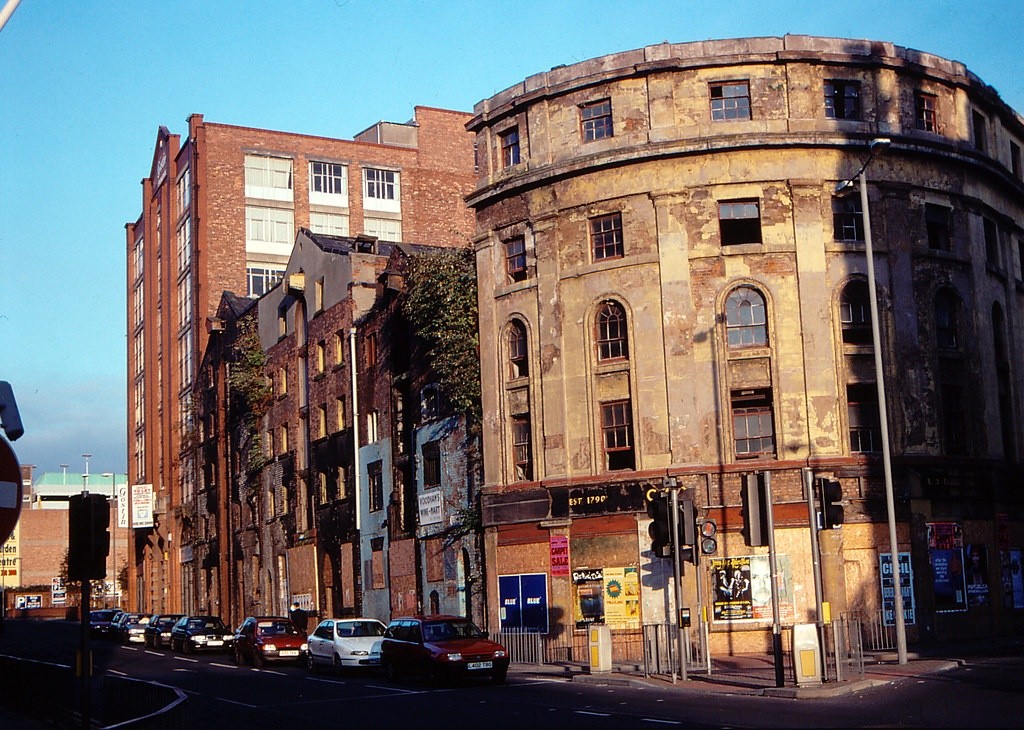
[647,493,671,557]
[699,518,718,556]
[65,494,111,582]
[817,477,844,530]
[740,474,769,547]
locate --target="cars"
[170,615,235,656]
[145,616,186,651]
[380,615,510,687]
[233,616,308,668]
[307,618,392,677]
[89,608,152,644]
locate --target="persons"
[290,602,309,633]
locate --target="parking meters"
[678,608,691,629]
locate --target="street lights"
[835,139,912,664]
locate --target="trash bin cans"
[588,625,612,675]
[791,623,822,688]
